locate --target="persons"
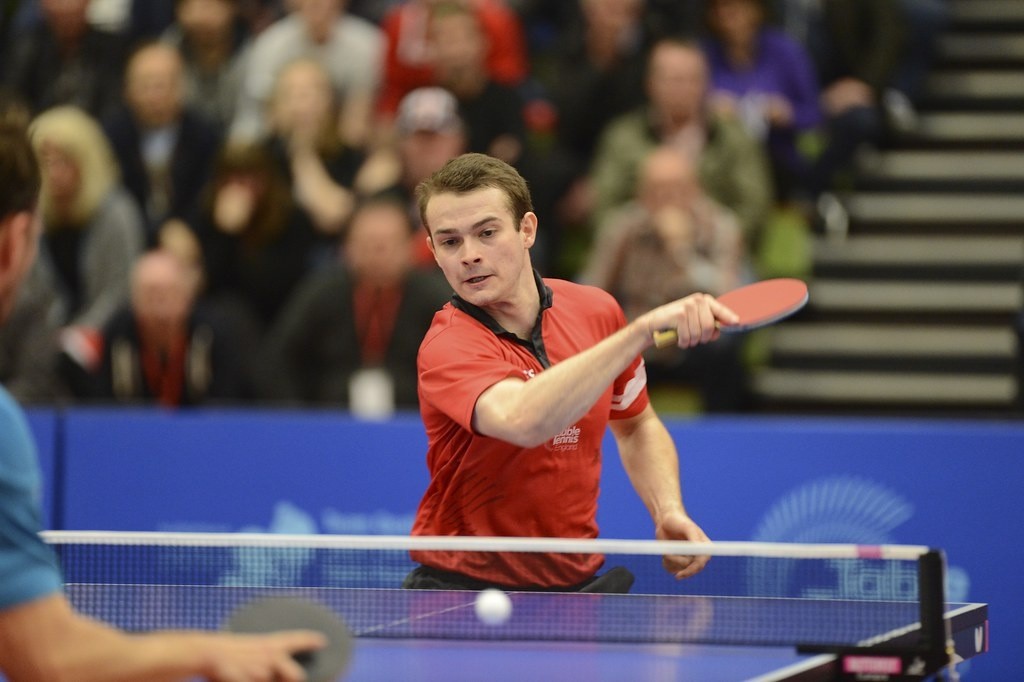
[0,116,328,682]
[402,152,739,592]
[0,1,928,421]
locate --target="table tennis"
[474,588,515,628]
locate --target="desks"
[0,527,994,682]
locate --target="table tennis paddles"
[652,276,809,350]
[221,596,352,682]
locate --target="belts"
[420,564,593,592]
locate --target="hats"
[397,87,458,134]
